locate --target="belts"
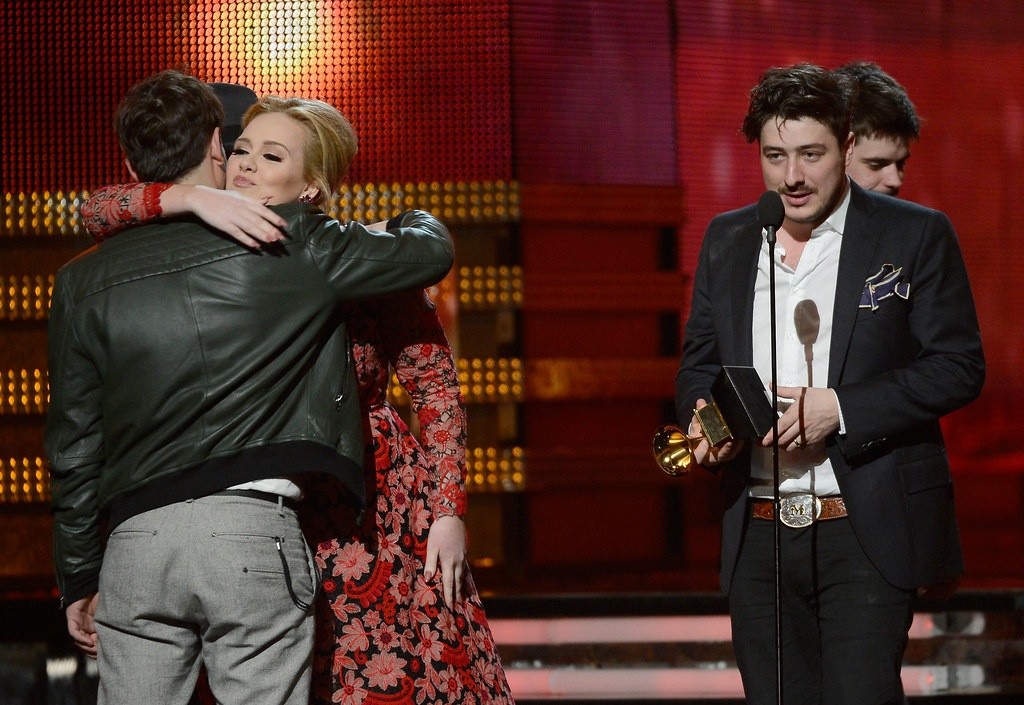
[215,486,300,518]
[746,494,848,529]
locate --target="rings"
[793,438,801,448]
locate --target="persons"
[45,69,457,705]
[672,59,986,705]
[82,92,514,705]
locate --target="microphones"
[759,191,786,243]
